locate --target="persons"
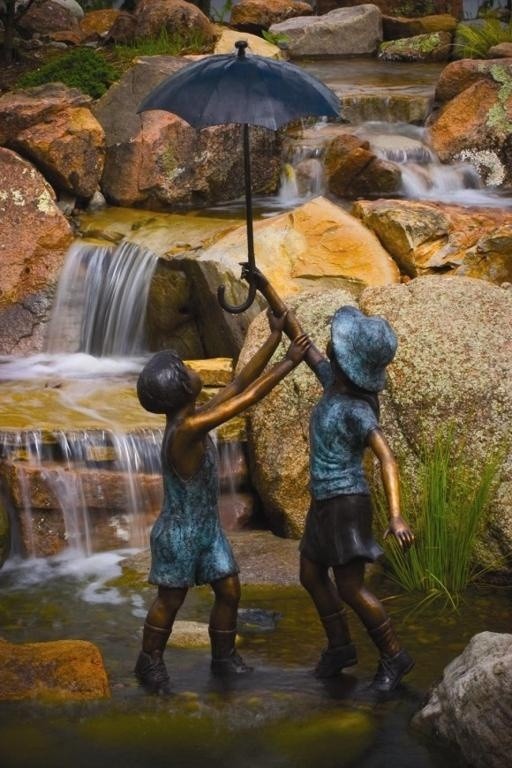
[134,303,314,698]
[233,258,417,697]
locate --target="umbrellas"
[135,37,349,318]
